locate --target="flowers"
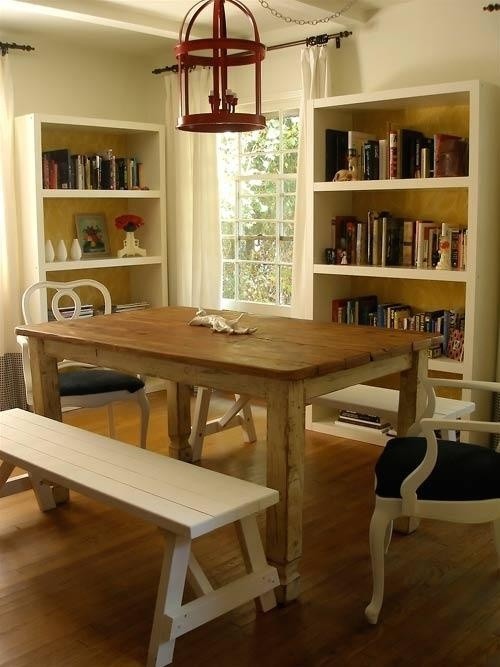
[116,215,146,231]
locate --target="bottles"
[70,239,82,260]
[57,240,67,261]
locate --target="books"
[334,410,461,442]
[331,208,468,269]
[49,305,96,320]
[332,295,465,362]
[42,148,143,189]
[325,120,469,182]
[111,302,150,313]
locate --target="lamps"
[173,0,268,132]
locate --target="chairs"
[362,379,500,627]
[14,279,151,451]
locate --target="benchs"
[0,406,278,667]
[190,382,475,465]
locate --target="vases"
[69,238,83,262]
[117,231,146,257]
[56,239,68,262]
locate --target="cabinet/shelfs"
[14,113,170,309]
[300,81,498,446]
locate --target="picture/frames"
[74,212,113,258]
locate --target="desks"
[16,304,443,602]
